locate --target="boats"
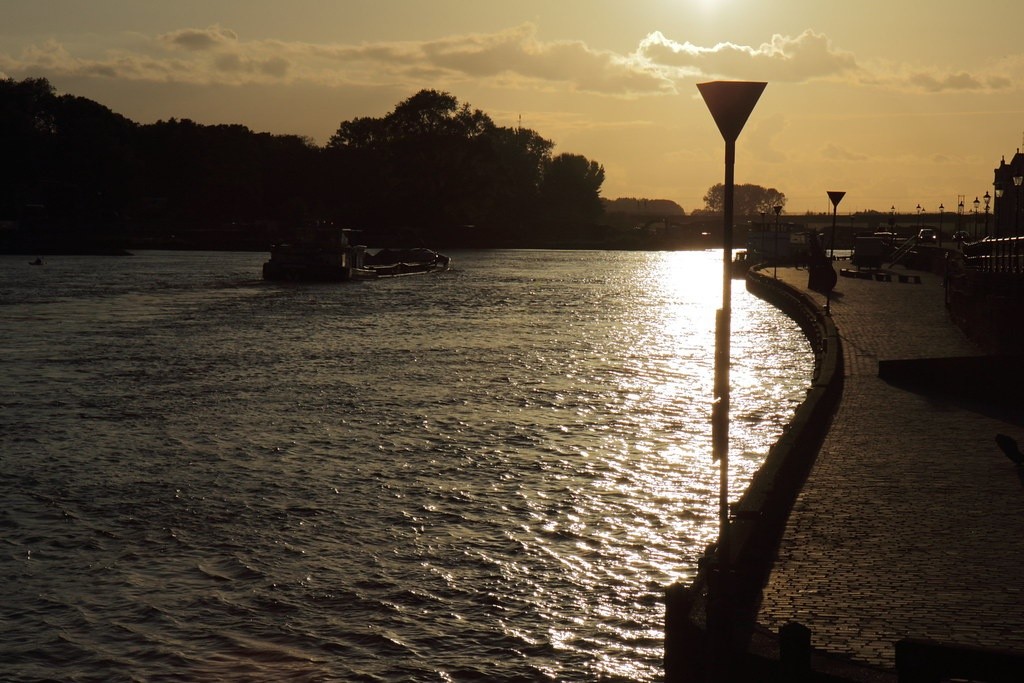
[265,217,452,283]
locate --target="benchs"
[899,273,921,285]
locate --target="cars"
[953,231,970,242]
[918,229,938,243]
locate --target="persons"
[35,258,41,263]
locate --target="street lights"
[995,185,1004,239]
[983,191,991,233]
[891,205,896,240]
[826,192,846,311]
[774,206,783,278]
[698,82,769,575]
[973,197,980,241]
[1013,172,1023,237]
[916,204,921,250]
[958,201,964,231]
[762,211,766,265]
[922,208,925,228]
[939,204,944,254]
